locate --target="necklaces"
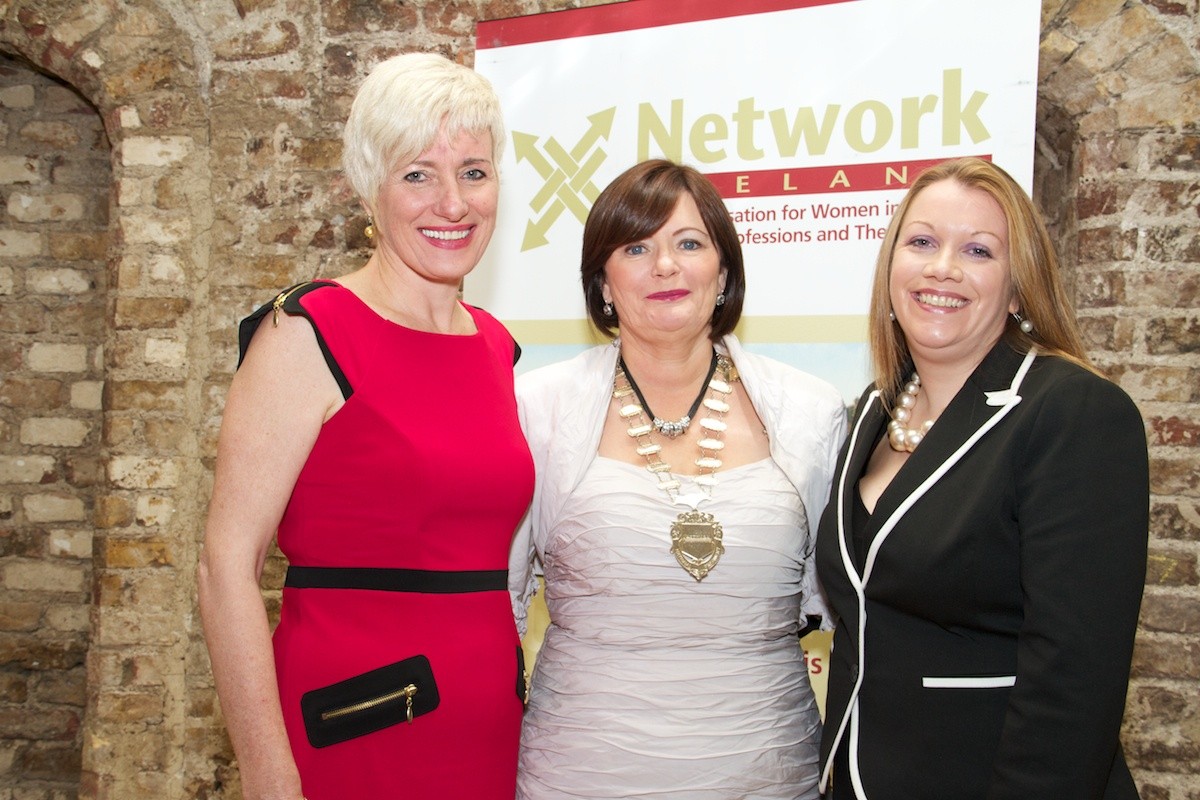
[887,372,935,453]
[613,353,743,583]
[620,345,718,439]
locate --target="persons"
[198,52,534,800]
[820,158,1149,800]
[508,159,846,800]
